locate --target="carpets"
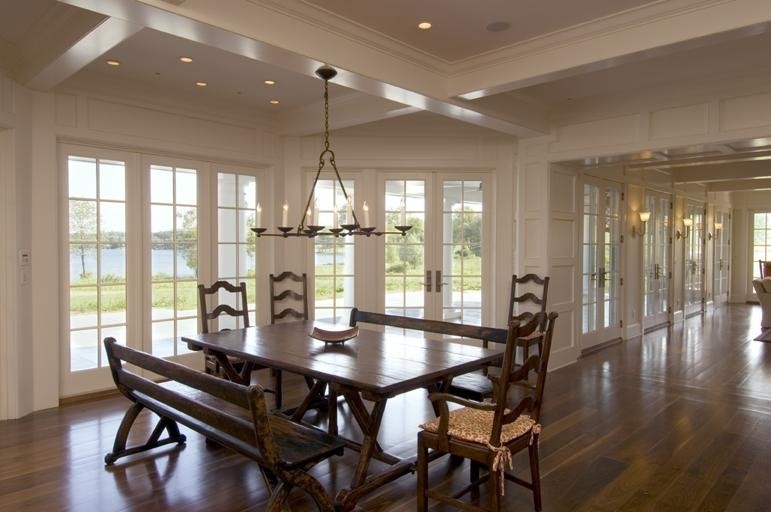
[753,327,771,342]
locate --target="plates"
[308,326,360,343]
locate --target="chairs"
[752,276,771,328]
[759,259,771,279]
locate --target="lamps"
[708,222,722,240]
[250,62,414,239]
[632,210,652,237]
[676,218,694,239]
